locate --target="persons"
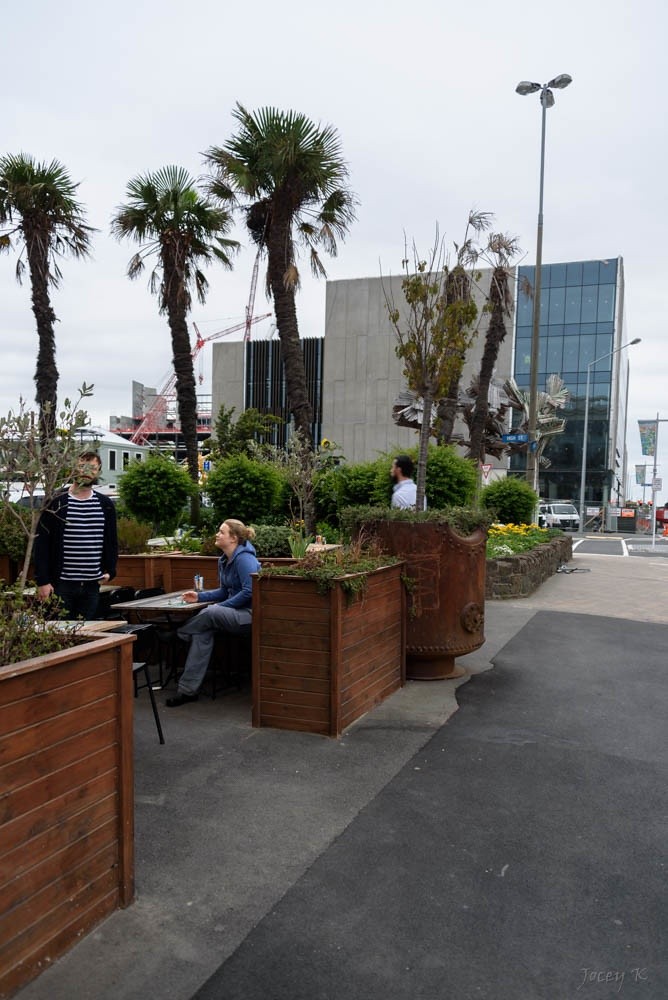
[33,453,118,621]
[390,455,427,513]
[662,503,668,537]
[160,519,262,707]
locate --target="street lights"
[578,337,642,535]
[516,73,573,488]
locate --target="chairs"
[35,588,253,744]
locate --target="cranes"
[128,251,272,445]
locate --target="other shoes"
[158,631,189,656]
[165,691,198,706]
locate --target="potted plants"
[338,503,500,681]
[250,549,410,738]
[0,573,138,1000]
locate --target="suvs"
[538,500,580,531]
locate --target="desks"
[35,620,128,632]
[111,589,219,690]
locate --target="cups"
[194,576,203,592]
